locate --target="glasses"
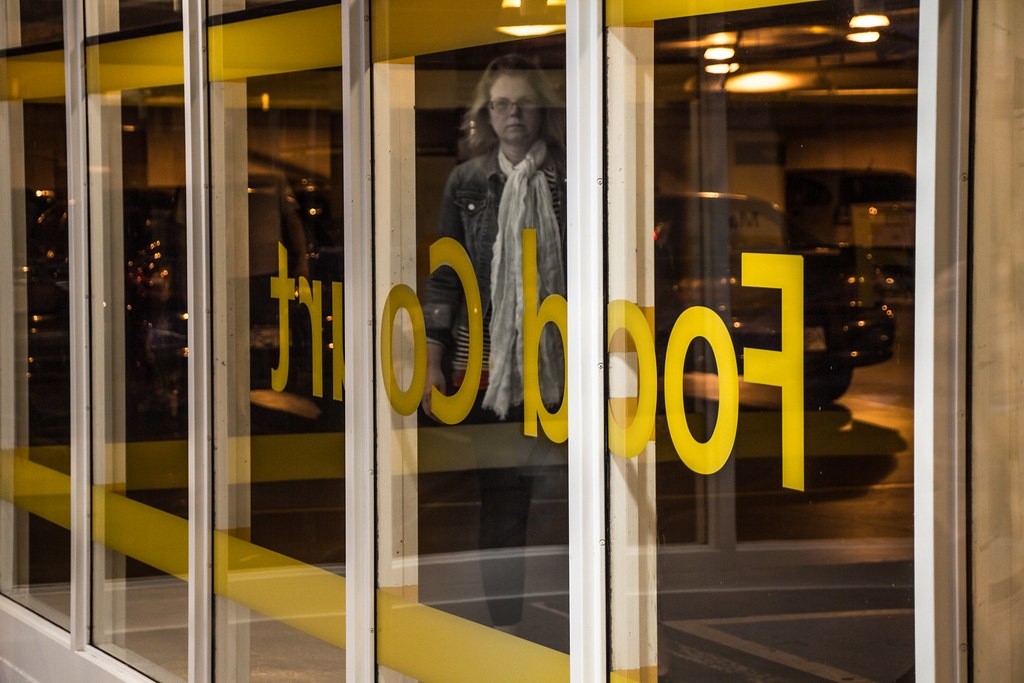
[488,94,538,116]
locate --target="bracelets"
[423,340,446,351]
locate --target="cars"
[26,177,345,436]
[654,193,899,413]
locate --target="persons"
[416,52,567,637]
